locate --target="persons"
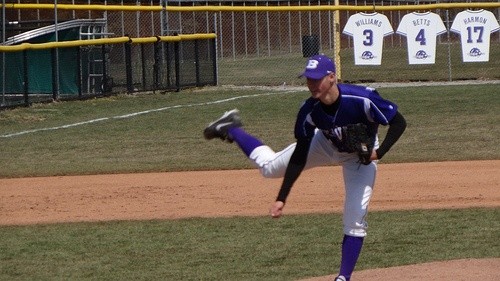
[203,54,407,281]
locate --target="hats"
[297,55,336,80]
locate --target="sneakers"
[334,273,350,281]
[203,108,242,141]
